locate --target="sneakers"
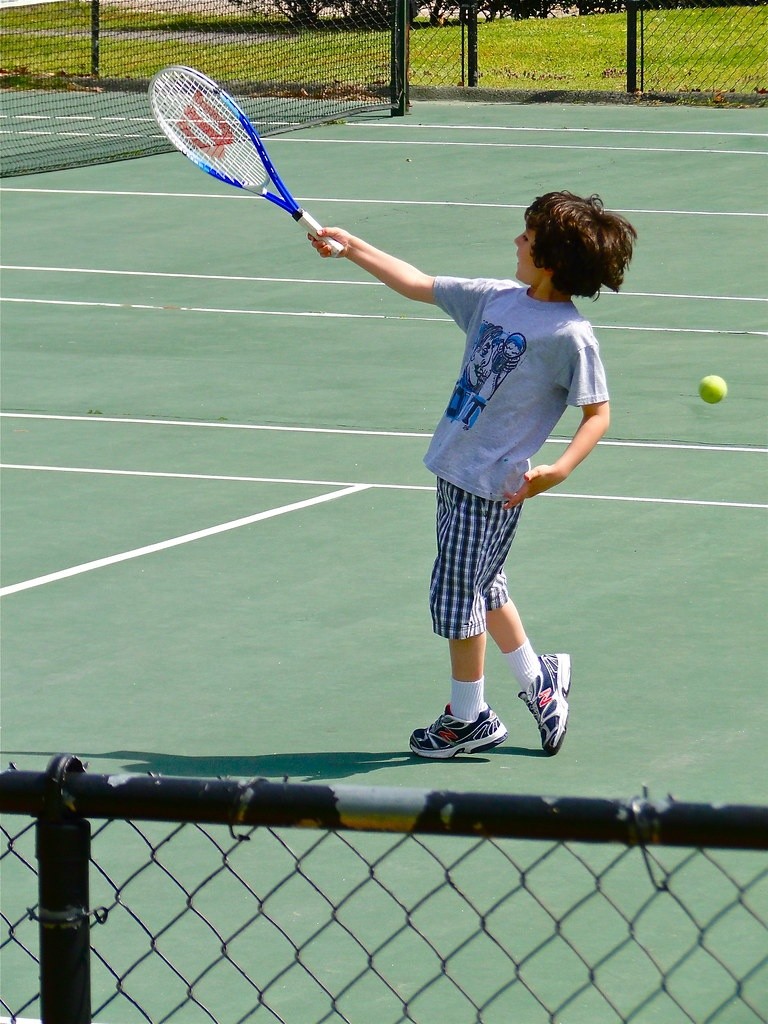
[408,701,510,759]
[519,650,571,757]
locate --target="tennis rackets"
[148,62,344,257]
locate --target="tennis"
[699,375,727,404]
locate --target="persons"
[307,190,639,758]
